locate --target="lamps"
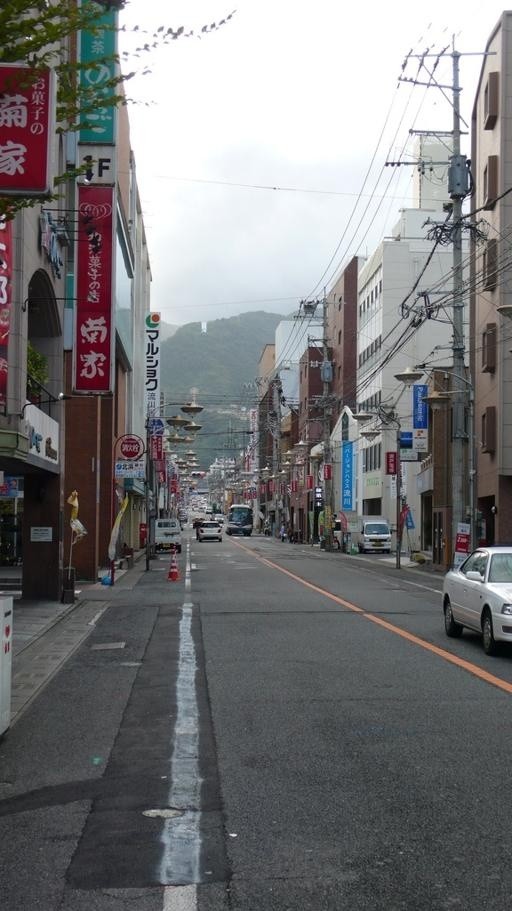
[55,167,96,186]
[20,391,76,419]
[22,293,98,312]
[41,205,101,254]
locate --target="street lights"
[353,408,399,570]
[393,365,477,553]
[144,402,200,573]
[253,439,318,549]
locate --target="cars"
[443,546,512,657]
[191,495,213,514]
[197,521,223,543]
[192,516,206,529]
[210,514,225,525]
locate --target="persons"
[262,521,271,535]
[280,525,287,544]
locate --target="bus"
[226,505,254,538]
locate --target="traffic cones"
[167,550,181,581]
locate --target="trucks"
[359,522,392,555]
